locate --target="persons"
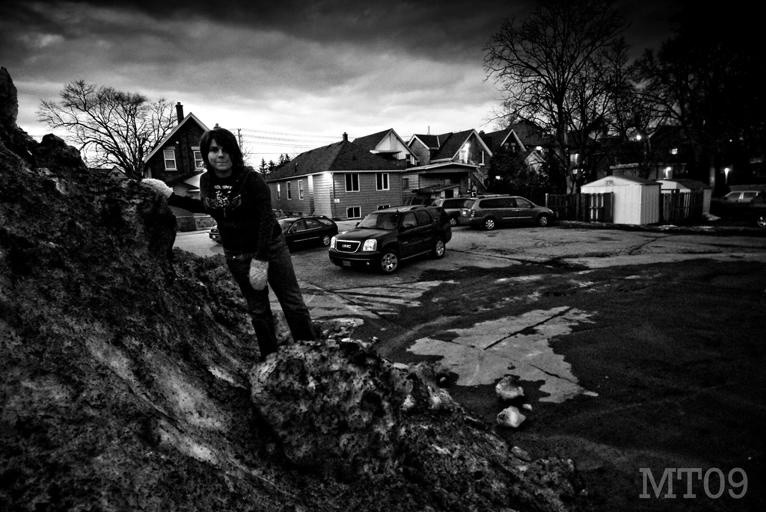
[137,126,315,361]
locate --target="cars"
[432,196,554,231]
[710,190,766,228]
[278,215,338,253]
[209,222,223,244]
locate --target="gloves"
[247,258,271,290]
[139,178,174,199]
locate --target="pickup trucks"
[329,205,452,275]
